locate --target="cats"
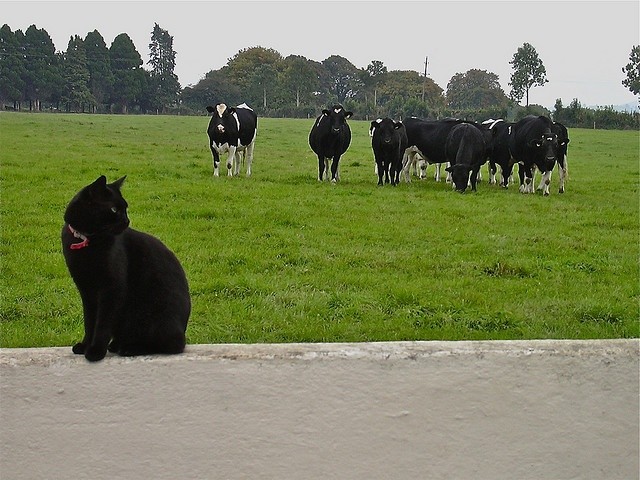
[61,171,191,363]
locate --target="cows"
[370,117,409,187]
[553,121,570,193]
[412,153,431,180]
[308,103,352,183]
[508,116,559,197]
[369,117,391,183]
[206,102,258,178]
[483,117,514,188]
[445,122,487,193]
[402,117,493,184]
